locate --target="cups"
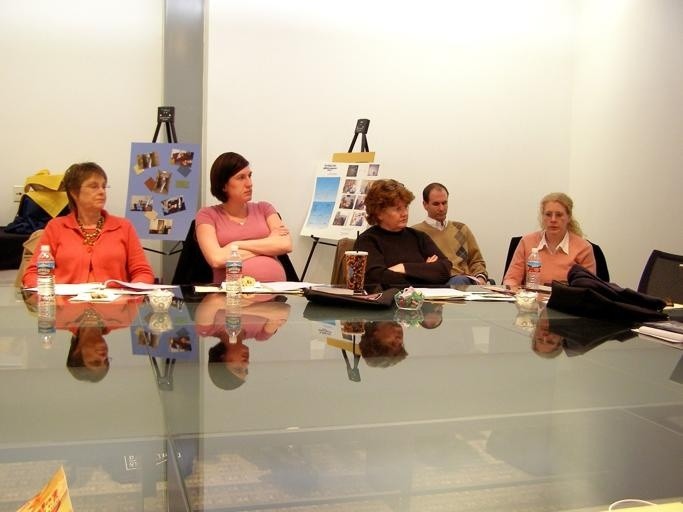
[344,250,369,296]
[339,319,366,336]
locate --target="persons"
[193,152,294,286]
[193,294,291,390]
[31,292,144,383]
[359,320,409,367]
[352,180,452,286]
[418,303,443,329]
[412,183,488,285]
[22,162,156,289]
[531,309,565,358]
[502,192,597,285]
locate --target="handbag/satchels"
[545,280,669,323]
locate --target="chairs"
[170,219,300,285]
[637,249,683,304]
[503,237,611,282]
[330,239,364,283]
[14,227,44,287]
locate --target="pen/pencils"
[481,296,513,298]
[472,292,495,295]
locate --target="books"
[22,279,179,296]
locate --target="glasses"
[383,205,409,212]
[85,357,112,367]
[78,183,110,192]
[380,341,404,349]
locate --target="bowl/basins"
[393,309,424,322]
[147,294,175,312]
[608,499,655,510]
[513,312,539,327]
[394,291,425,311]
[515,294,537,310]
[147,312,173,330]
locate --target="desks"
[1,280,683,512]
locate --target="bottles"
[37,301,57,351]
[526,248,542,291]
[226,297,242,345]
[36,244,57,302]
[224,244,243,298]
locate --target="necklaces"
[78,217,104,245]
[77,307,105,332]
